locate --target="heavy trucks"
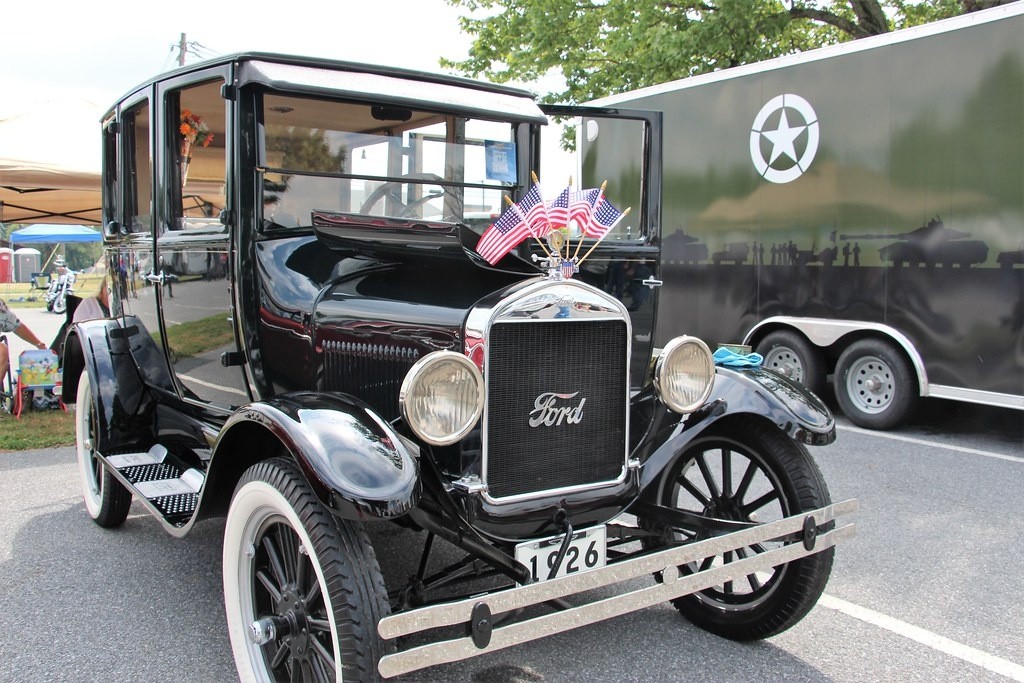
[573,0,1024,436]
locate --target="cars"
[56,46,844,682]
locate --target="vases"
[180,138,192,186]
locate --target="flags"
[475,184,625,266]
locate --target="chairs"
[13,349,67,419]
[271,176,339,224]
[30,273,52,300]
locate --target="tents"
[10,224,103,284]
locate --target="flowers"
[179,109,214,148]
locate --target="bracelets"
[37,343,46,347]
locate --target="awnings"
[0,157,341,226]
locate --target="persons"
[0,297,48,400]
[207,247,230,282]
[141,253,154,287]
[577,302,591,312]
